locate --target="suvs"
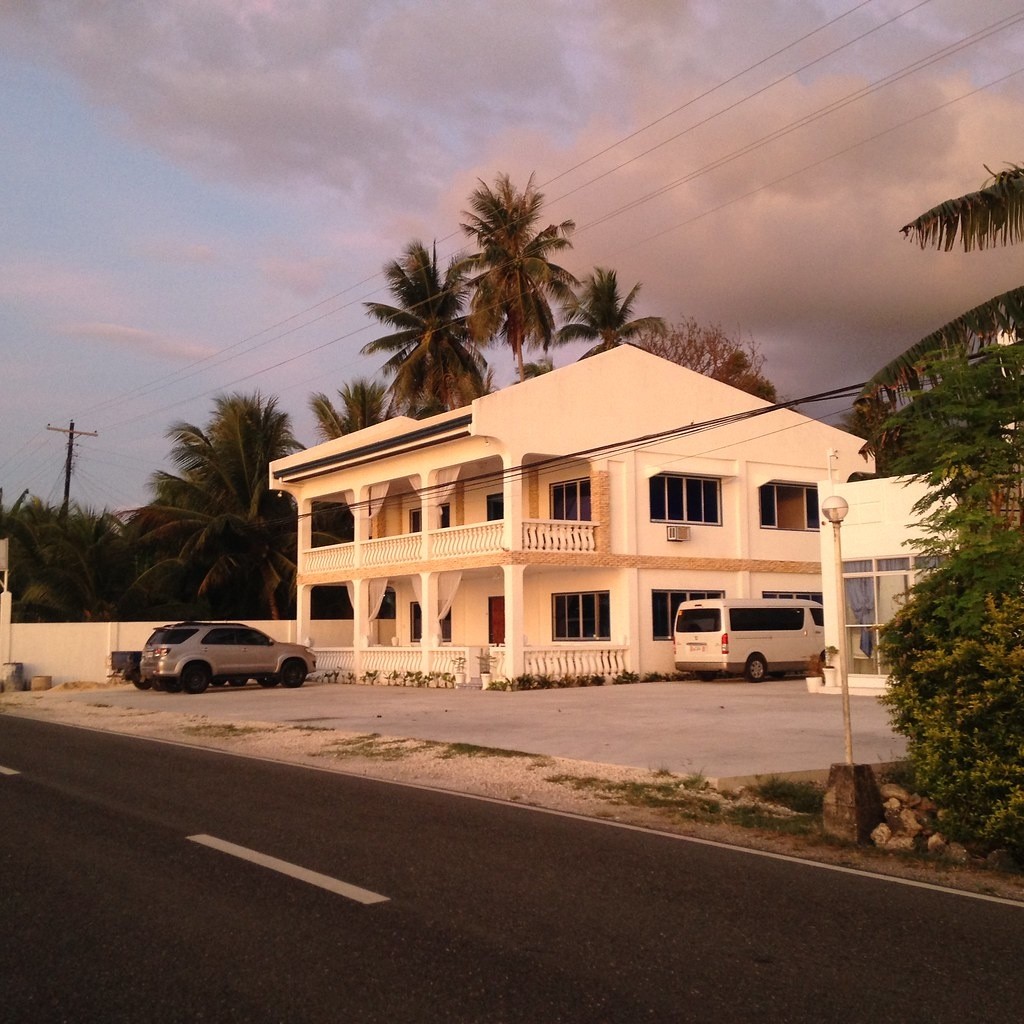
[140,621,320,695]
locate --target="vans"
[673,597,823,684]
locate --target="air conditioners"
[667,525,692,541]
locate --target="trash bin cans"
[110,649,141,673]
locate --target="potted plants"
[477,652,496,689]
[805,645,838,687]
[450,657,466,684]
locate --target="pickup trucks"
[111,648,152,692]
